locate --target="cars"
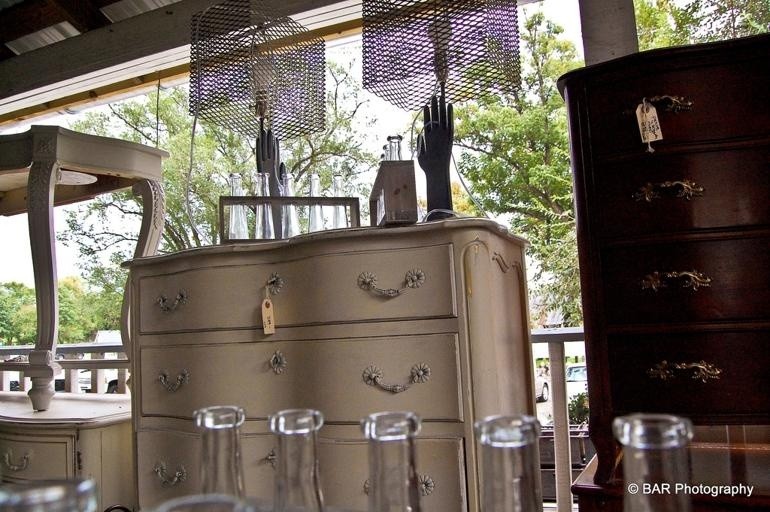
[531,364,589,408]
[1,328,133,393]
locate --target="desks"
[0,123,169,412]
[567,441,770,512]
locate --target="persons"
[256,129,287,240]
[417,95,454,221]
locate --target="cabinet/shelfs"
[553,30,769,486]
[2,391,136,512]
[119,216,542,512]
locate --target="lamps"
[359,0,524,222]
[185,0,345,241]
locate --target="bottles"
[377,133,403,227]
[228,171,346,241]
[194,404,247,502]
[268,407,324,512]
[612,413,693,512]
[470,415,544,511]
[363,410,423,511]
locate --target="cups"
[151,493,265,511]
[0,475,97,512]
